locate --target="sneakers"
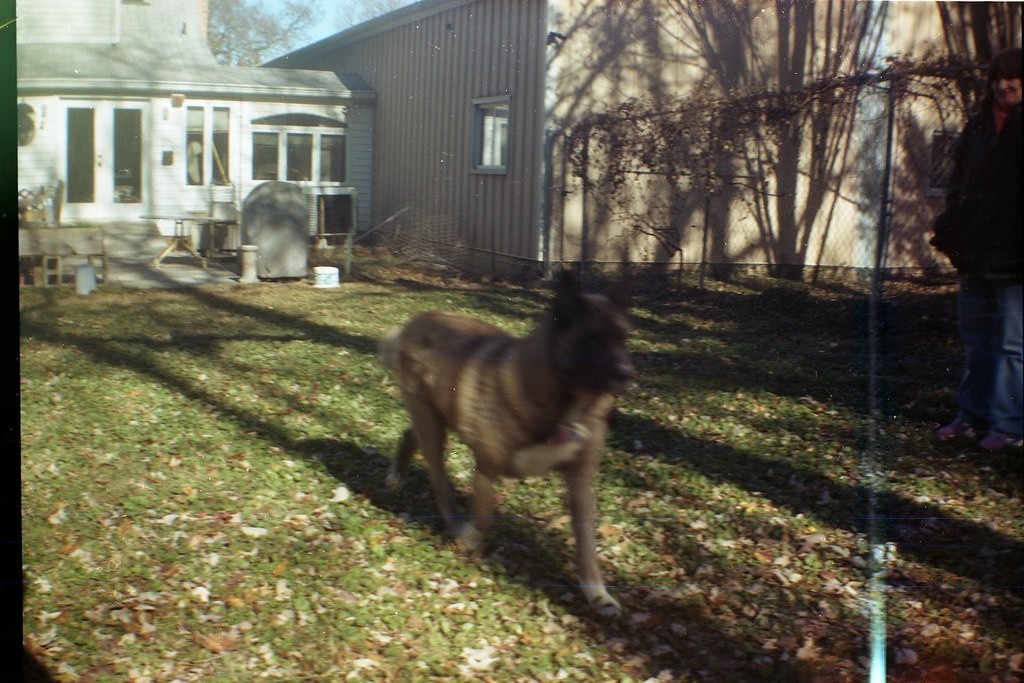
[979,431,1023,450]
[936,419,977,440]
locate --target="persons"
[928,47,1024,452]
[188,137,203,186]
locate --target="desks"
[140,215,215,269]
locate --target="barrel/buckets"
[313,267,339,287]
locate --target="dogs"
[373,265,640,619]
